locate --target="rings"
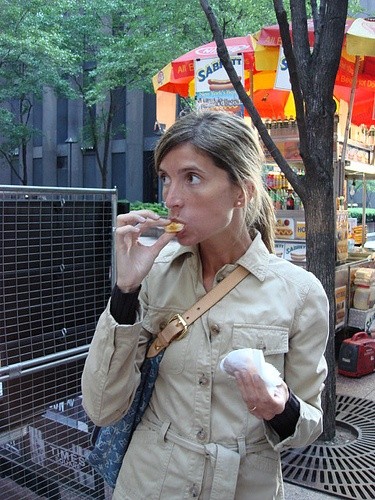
[248,406,257,412]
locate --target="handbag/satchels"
[85,265,250,488]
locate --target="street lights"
[64,136,79,188]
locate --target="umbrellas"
[152,34,341,123]
[250,16,375,163]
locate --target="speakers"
[338,332,375,378]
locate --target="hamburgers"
[272,226,294,239]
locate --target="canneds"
[265,173,290,189]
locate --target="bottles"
[267,173,288,200]
[336,195,346,210]
[280,187,301,210]
[355,124,375,145]
[264,115,298,136]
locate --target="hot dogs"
[207,77,243,91]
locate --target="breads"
[207,105,243,112]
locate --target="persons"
[80,111,331,500]
